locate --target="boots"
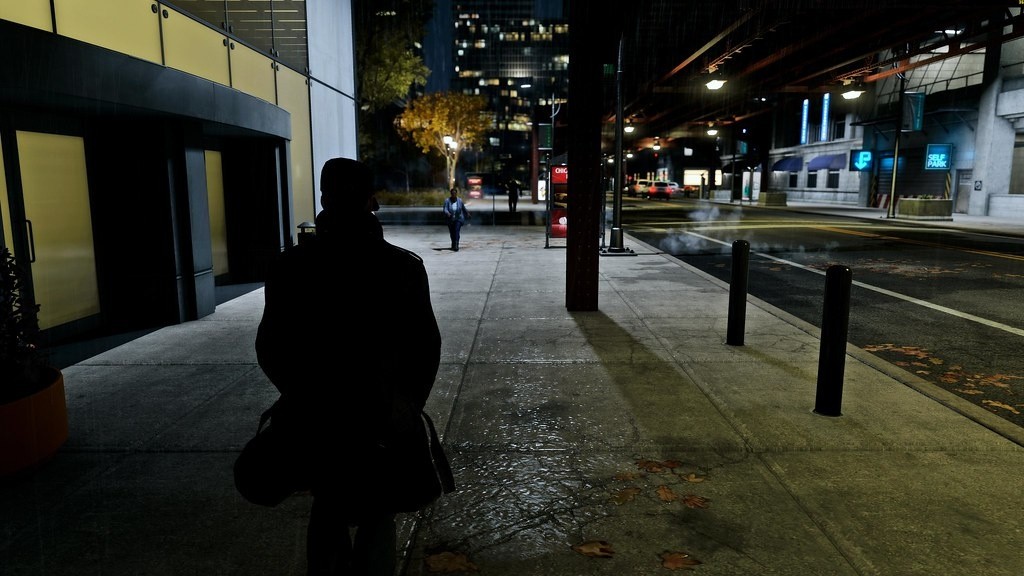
[452,241,459,251]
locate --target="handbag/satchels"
[235,409,295,507]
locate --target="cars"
[629,179,649,197]
[642,181,669,200]
[668,182,678,189]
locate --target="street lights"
[443,134,458,190]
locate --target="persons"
[508,179,523,212]
[443,189,471,252]
[254,158,442,576]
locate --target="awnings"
[772,154,846,171]
[851,108,978,143]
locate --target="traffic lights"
[654,152,659,159]
